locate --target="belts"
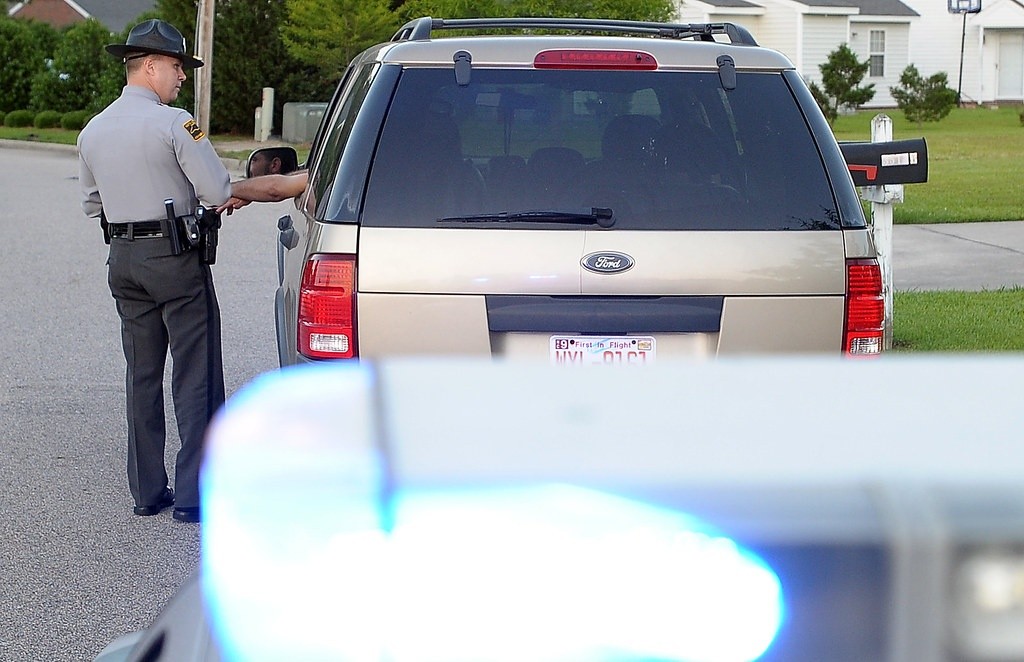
[112,225,162,238]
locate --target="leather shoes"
[134,486,174,515]
[173,506,200,522]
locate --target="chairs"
[389,108,760,228]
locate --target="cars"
[94,346,1022,658]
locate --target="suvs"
[243,15,896,369]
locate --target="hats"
[104,18,205,67]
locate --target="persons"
[216,169,309,213]
[76,20,234,522]
[250,152,294,177]
